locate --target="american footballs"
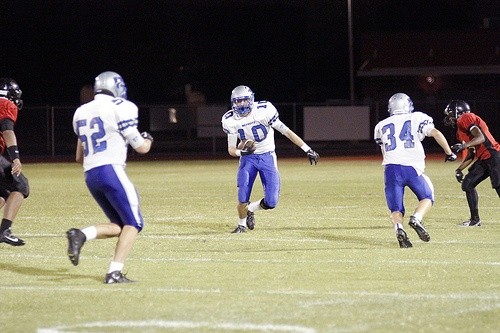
[237,138,256,155]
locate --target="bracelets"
[301,144,311,152]
[235,150,241,156]
[7,145,19,162]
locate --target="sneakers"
[395,222,413,248]
[104,270,140,284]
[232,225,247,233]
[408,215,430,242]
[455,217,482,227]
[246,201,255,230]
[0,229,26,246]
[66,228,86,266]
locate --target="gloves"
[237,140,257,156]
[140,131,154,142]
[305,148,321,166]
[452,143,465,153]
[444,153,457,162]
[455,169,464,183]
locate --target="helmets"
[231,85,255,115]
[445,100,471,127]
[94,71,127,100]
[388,93,414,115]
[0,77,23,111]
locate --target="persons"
[444,101,500,226]
[66,72,153,284]
[0,81,29,246]
[374,93,457,248]
[221,86,320,233]
